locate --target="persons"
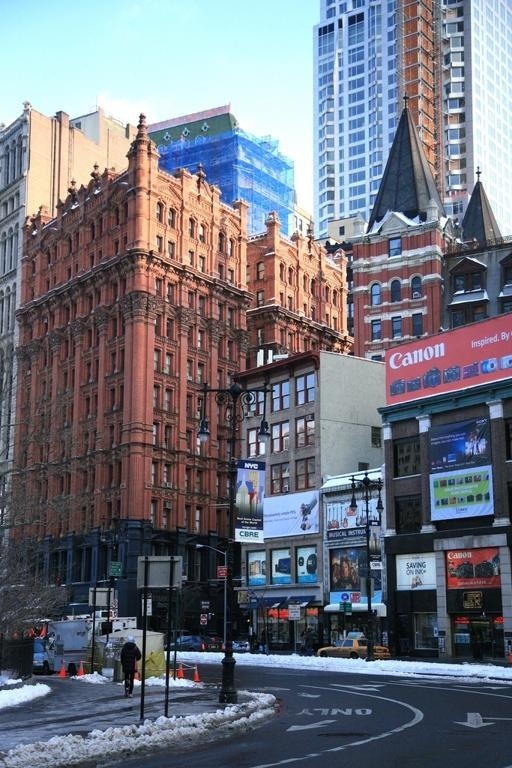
[259,628,271,651]
[332,557,340,582]
[462,427,488,461]
[332,554,359,589]
[120,634,142,697]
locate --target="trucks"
[48,616,137,676]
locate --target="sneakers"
[125,687,134,698]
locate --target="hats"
[128,636,135,643]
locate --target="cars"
[317,638,391,660]
[34,640,54,675]
[164,635,226,652]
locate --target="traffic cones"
[59,664,66,678]
[177,662,184,678]
[78,661,84,676]
[134,671,139,680]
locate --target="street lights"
[194,374,275,702]
[348,471,383,661]
